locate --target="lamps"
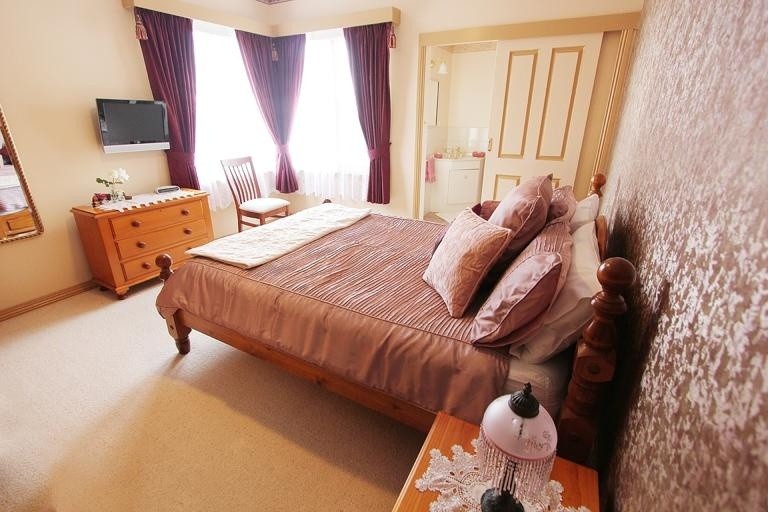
[478,379,560,511]
[435,59,448,74]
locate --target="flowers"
[97,167,129,202]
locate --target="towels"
[426,159,435,182]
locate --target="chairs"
[219,156,291,233]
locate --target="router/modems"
[155,185,179,193]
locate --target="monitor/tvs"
[96,97,171,155]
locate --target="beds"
[156,173,637,463]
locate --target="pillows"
[546,185,575,224]
[570,194,599,233]
[509,221,603,365]
[470,222,573,346]
[488,174,551,258]
[423,206,511,319]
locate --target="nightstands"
[392,410,600,512]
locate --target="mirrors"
[0,111,43,244]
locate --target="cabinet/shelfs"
[69,188,215,302]
[448,160,479,204]
